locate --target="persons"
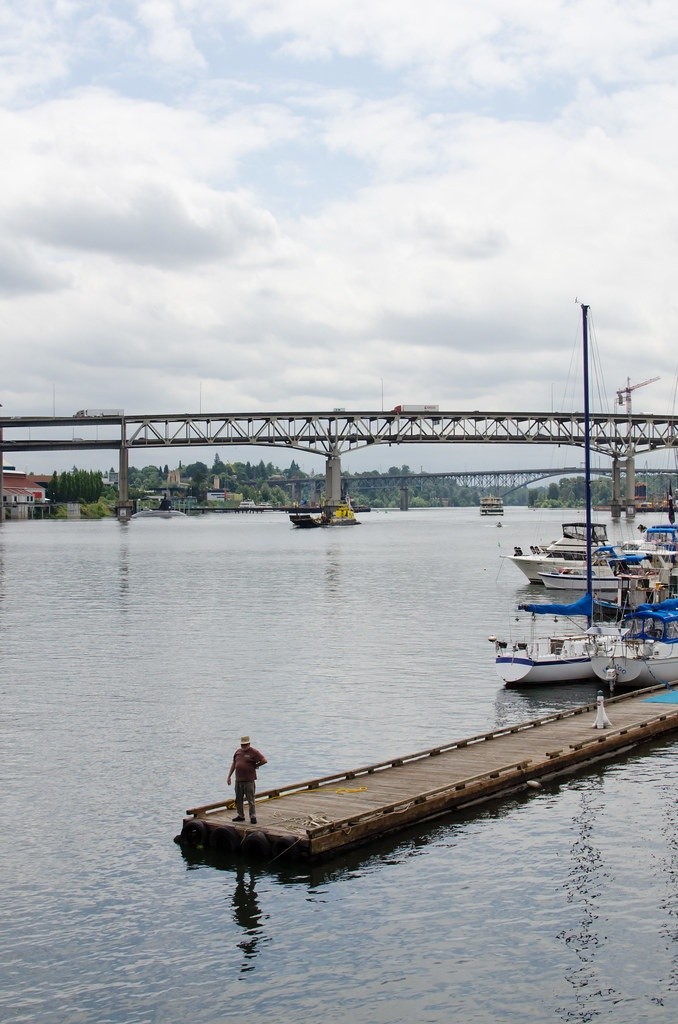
[227,736,267,823]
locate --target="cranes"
[616,376,662,413]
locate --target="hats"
[240,736,250,744]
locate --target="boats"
[479,495,504,517]
[290,502,362,528]
[537,545,660,591]
[497,522,677,584]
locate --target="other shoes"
[251,818,257,824]
[232,816,245,821]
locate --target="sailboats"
[492,303,632,685]
[584,371,678,685]
